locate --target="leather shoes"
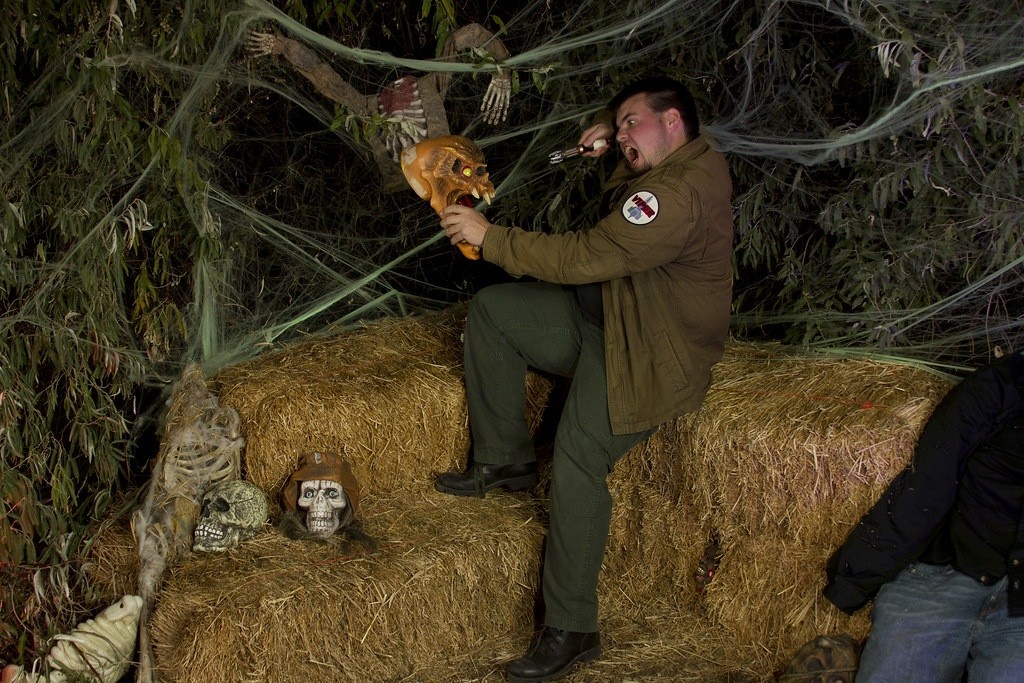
[436,459,535,496]
[509,623,601,682]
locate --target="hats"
[284,452,359,513]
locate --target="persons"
[823,352,1024,683]
[436,81,733,683]
